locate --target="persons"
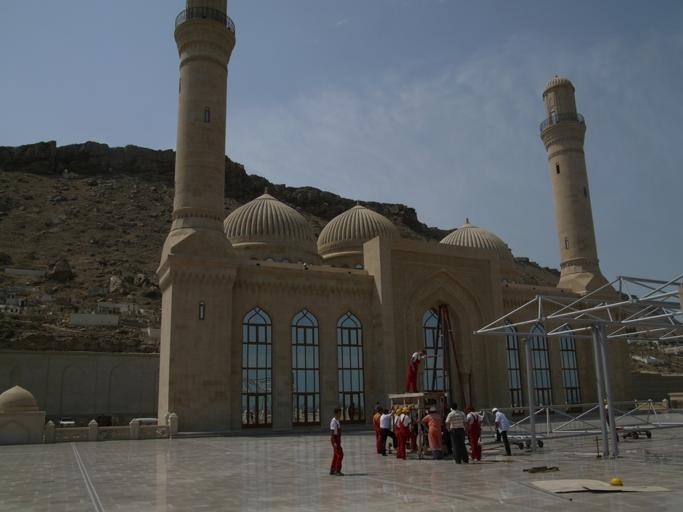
[329,408,344,476]
[405,349,427,392]
[371,400,483,464]
[604,398,620,442]
[491,406,512,456]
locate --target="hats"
[396,407,409,415]
[492,408,497,412]
